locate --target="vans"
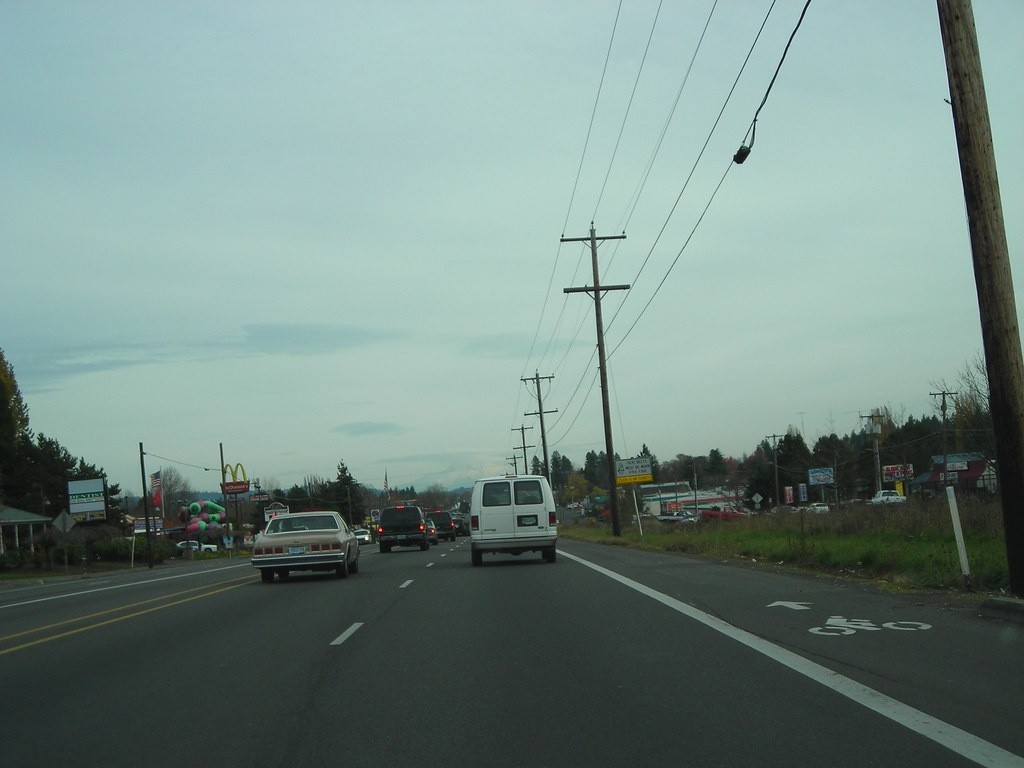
[459,475,558,567]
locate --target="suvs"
[425,511,457,542]
[378,506,431,553]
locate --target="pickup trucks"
[656,510,693,523]
[870,490,907,506]
[700,506,750,522]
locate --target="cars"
[353,529,372,545]
[251,510,359,584]
[739,505,808,515]
[426,520,440,546]
[450,513,470,537]
[806,502,830,514]
[176,540,217,554]
